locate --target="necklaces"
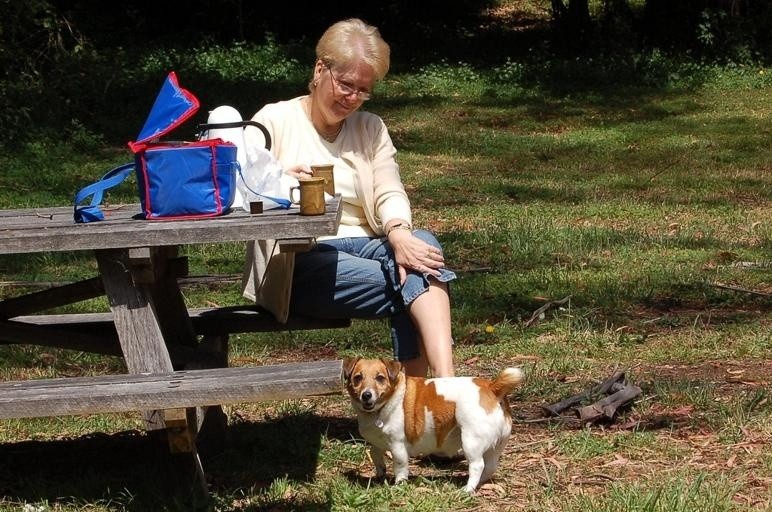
[310,118,344,142]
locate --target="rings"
[426,251,432,260]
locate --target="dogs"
[343,356,526,493]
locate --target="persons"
[239,18,463,378]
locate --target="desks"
[0,191,346,512]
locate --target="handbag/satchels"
[72,70,238,224]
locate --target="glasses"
[329,66,377,101]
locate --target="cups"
[302,163,337,198]
[291,176,324,219]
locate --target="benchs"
[0,297,353,403]
[0,355,346,460]
